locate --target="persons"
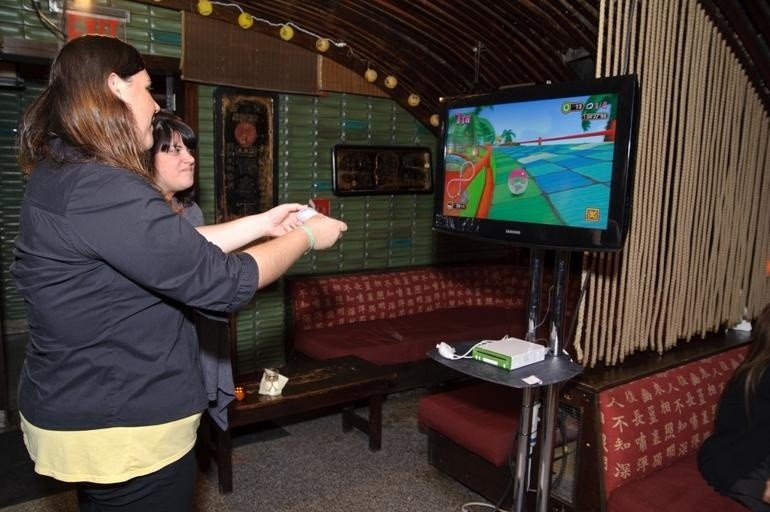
[11,31,349,510]
[135,109,235,462]
[696,302,770,512]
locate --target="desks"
[193,353,397,494]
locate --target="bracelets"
[299,225,315,255]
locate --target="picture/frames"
[331,143,434,199]
[212,85,281,294]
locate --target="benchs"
[285,260,669,402]
[416,385,549,512]
[576,327,762,512]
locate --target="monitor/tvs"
[432,75,641,252]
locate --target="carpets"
[1,420,292,510]
